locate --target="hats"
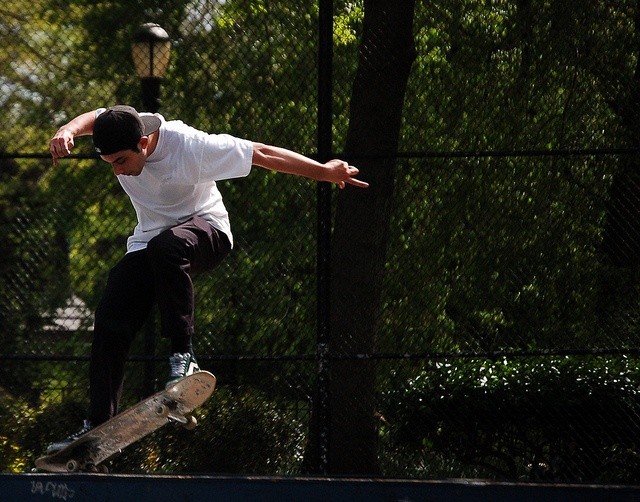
[93,106,161,154]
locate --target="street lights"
[130,22,173,397]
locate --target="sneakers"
[166,353,200,390]
[46,418,93,454]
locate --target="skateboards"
[34,372,216,475]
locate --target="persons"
[47,105,369,454]
